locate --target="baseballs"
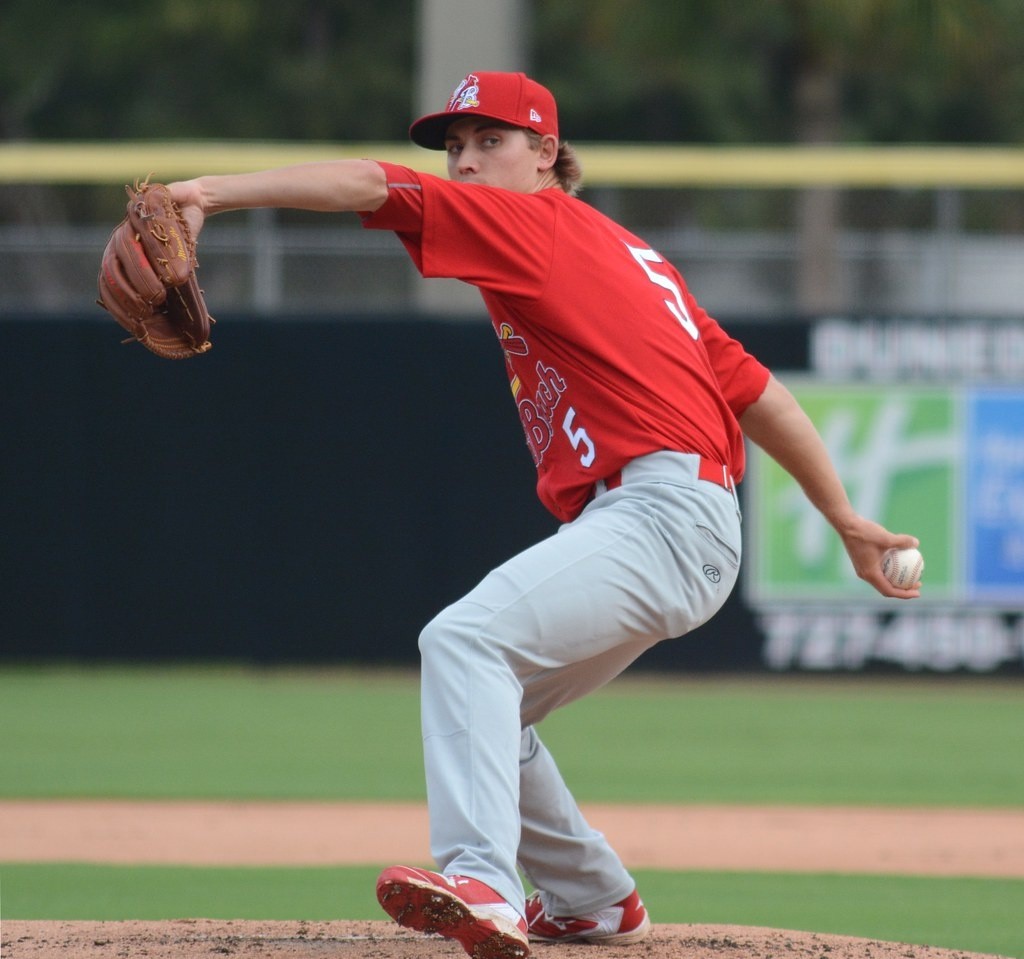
[876,542,930,595]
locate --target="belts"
[595,452,732,495]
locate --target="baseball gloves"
[98,181,211,360]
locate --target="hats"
[408,71,560,151]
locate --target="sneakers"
[377,865,531,959]
[523,885,652,941]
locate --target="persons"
[162,72,921,959]
